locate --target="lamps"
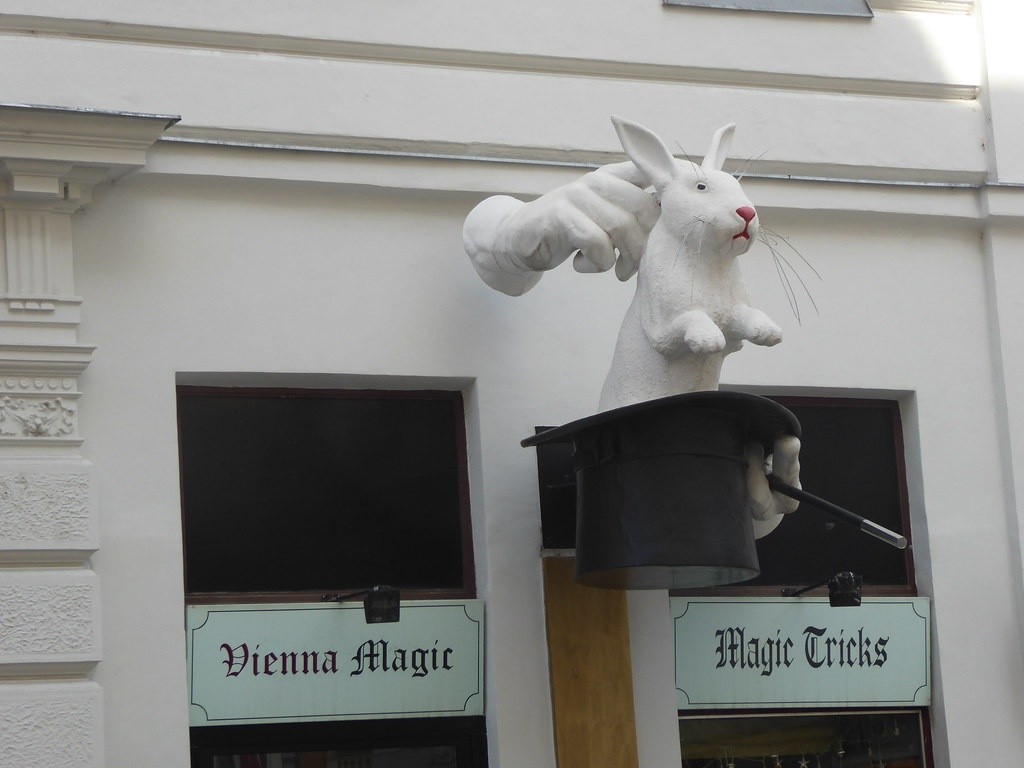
[780,571,862,607]
[319,581,400,623]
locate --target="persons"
[463,159,660,297]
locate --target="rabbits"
[596,113,823,414]
